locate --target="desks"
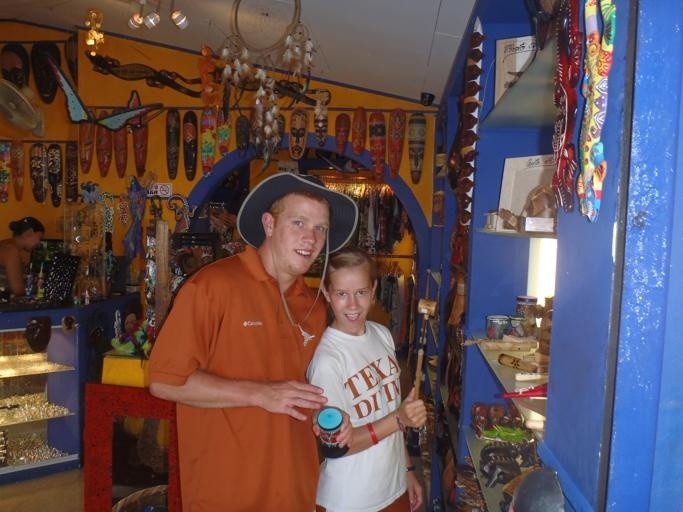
[83,383,181,512]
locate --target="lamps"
[128,0,145,30]
[170,0,188,31]
[84,8,104,56]
[143,0,164,29]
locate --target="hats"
[236,171,360,256]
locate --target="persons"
[2,214,47,308]
[302,248,428,512]
[143,171,358,511]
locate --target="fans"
[0,79,45,138]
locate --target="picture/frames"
[492,33,540,112]
[494,151,566,234]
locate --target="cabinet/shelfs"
[433,1,681,512]
[0,322,78,486]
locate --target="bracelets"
[404,464,416,472]
[363,423,379,446]
[394,408,407,432]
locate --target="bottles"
[515,295,537,318]
[486,314,510,339]
[317,406,344,450]
[509,313,529,338]
